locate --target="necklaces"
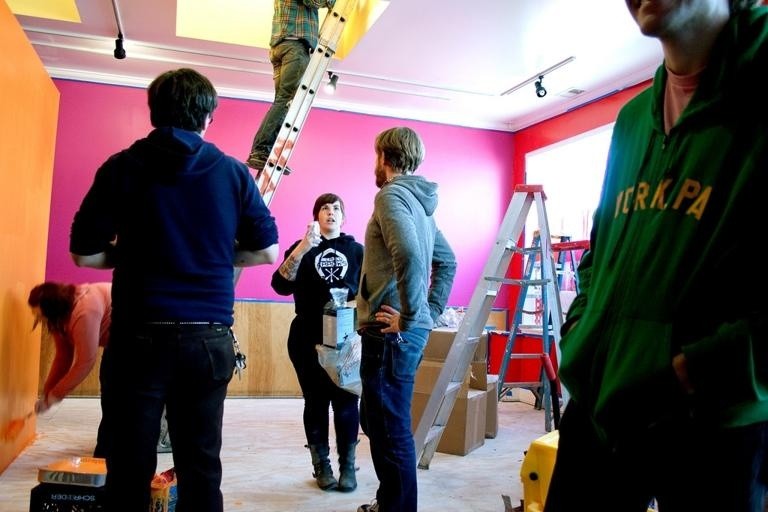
[380,175,397,189]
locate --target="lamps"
[534,75,546,98]
[326,72,340,92]
[113,33,126,59]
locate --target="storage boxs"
[409,326,498,458]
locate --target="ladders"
[402,188,562,471]
[497,230,581,431]
[222,2,352,285]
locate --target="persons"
[270,191,366,494]
[248,0,336,179]
[355,126,459,511]
[28,281,175,454]
[540,1,767,510]
[68,67,281,511]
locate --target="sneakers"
[244,153,292,176]
[358,503,379,512]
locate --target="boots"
[305,442,337,491]
[338,441,359,491]
